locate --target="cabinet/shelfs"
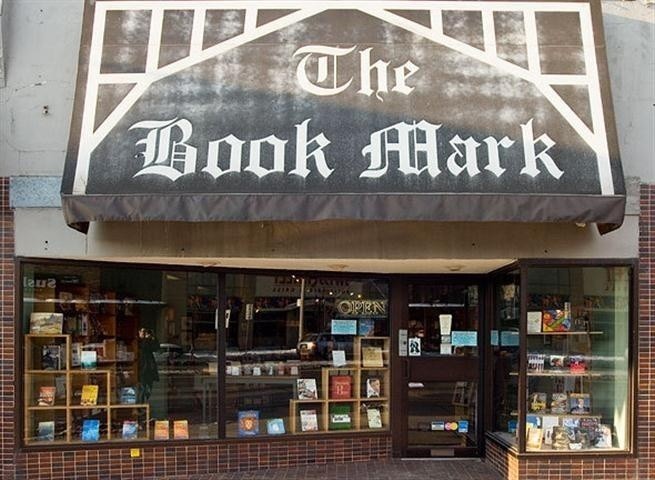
[287,336,391,434]
[508,330,606,420]
[23,331,150,445]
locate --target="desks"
[198,375,299,426]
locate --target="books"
[79,350,99,370]
[82,418,101,442]
[39,343,62,372]
[79,384,100,406]
[266,418,286,435]
[37,385,57,407]
[207,353,299,379]
[38,419,55,442]
[154,419,169,440]
[288,306,389,434]
[508,294,616,449]
[122,418,139,440]
[120,386,138,406]
[173,419,191,440]
[27,310,64,335]
[237,408,261,437]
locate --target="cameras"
[143,328,152,336]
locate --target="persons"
[136,323,163,406]
